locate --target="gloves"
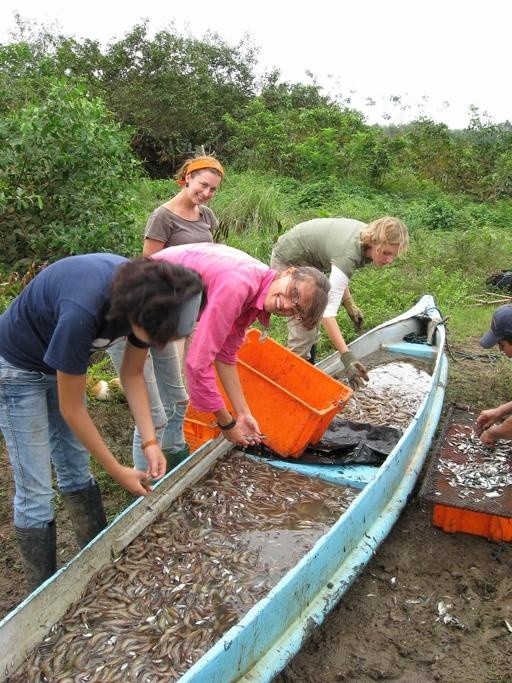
[340,350,369,390]
[341,297,365,333]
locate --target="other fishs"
[8,455,355,678]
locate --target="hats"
[480,304,512,349]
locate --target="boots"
[164,444,190,471]
[62,483,108,550]
[13,522,56,591]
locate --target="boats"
[0,294,449,683]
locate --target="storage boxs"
[212,327,355,458]
[432,423,511,542]
[184,397,221,456]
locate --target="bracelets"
[141,439,158,449]
[215,412,237,430]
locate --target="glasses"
[290,280,301,321]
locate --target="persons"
[142,155,224,366]
[476,304,512,448]
[107,242,330,485]
[0,253,208,594]
[271,216,408,391]
